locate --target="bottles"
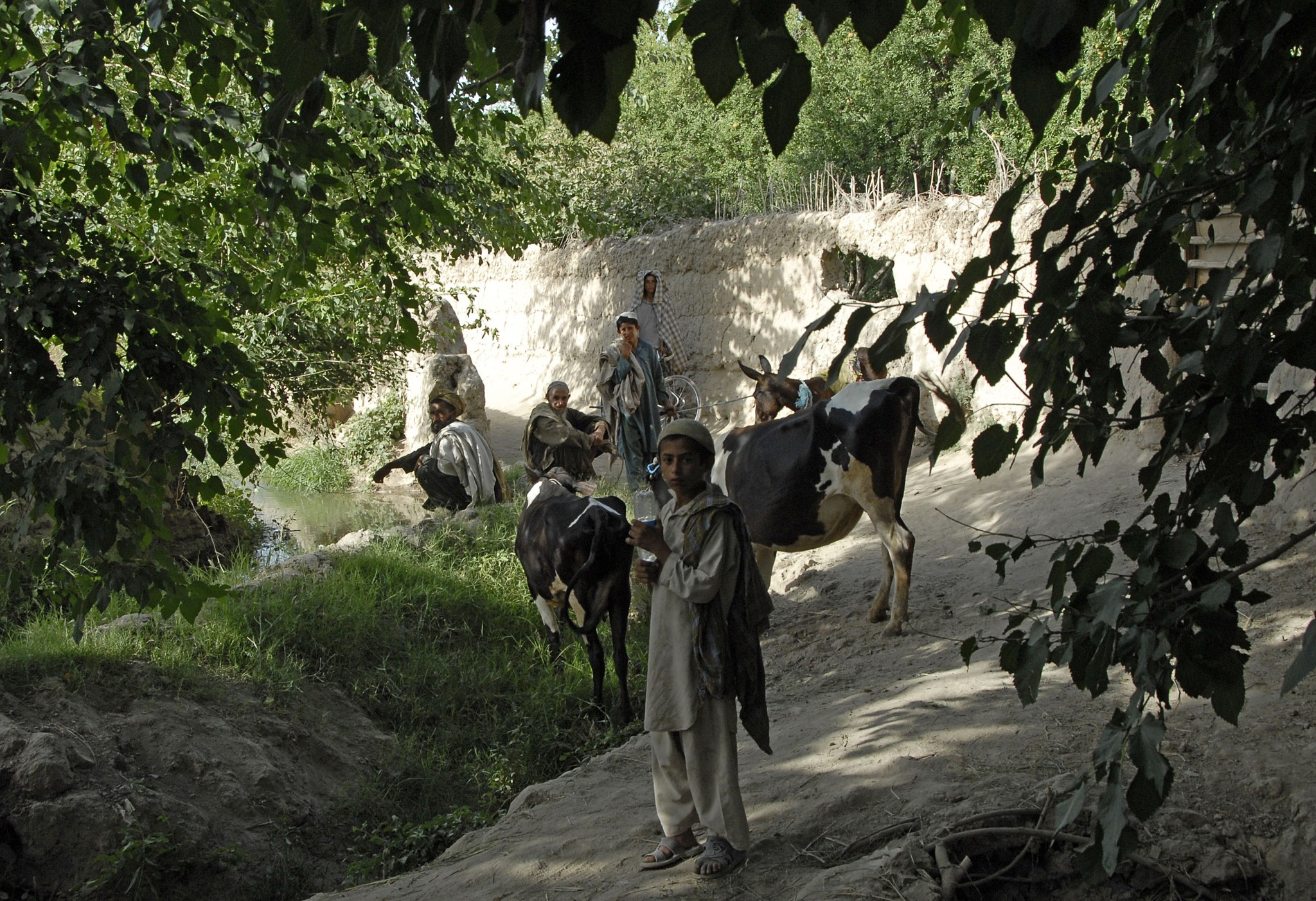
[634,481,657,562]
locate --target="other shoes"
[413,514,435,533]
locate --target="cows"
[514,464,636,722]
[711,375,966,638]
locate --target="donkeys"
[738,355,835,425]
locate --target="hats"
[656,418,716,455]
[615,311,638,325]
[429,390,467,417]
[547,381,569,392]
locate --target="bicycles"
[588,345,702,443]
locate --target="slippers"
[639,837,705,868]
[693,836,746,879]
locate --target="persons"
[521,381,616,479]
[625,418,773,879]
[631,267,672,374]
[373,381,507,512]
[597,311,676,493]
